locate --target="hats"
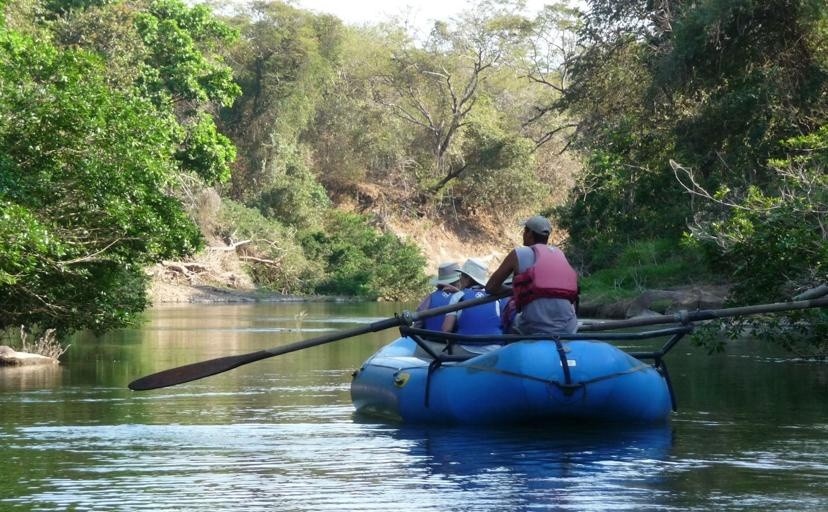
[520,216,553,235]
[431,261,460,285]
[453,258,488,287]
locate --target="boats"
[350,312,690,427]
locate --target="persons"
[414,258,515,360]
[485,217,578,334]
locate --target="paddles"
[128,291,517,391]
